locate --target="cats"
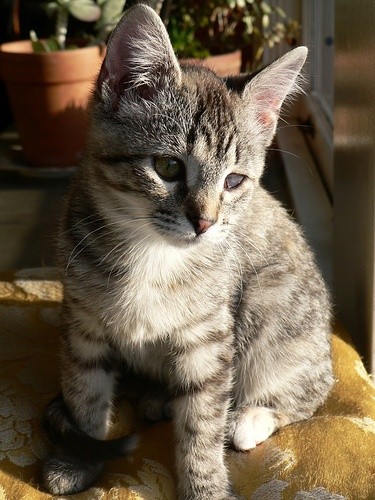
[42,3,336,500]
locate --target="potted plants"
[1,0,124,168]
[161,0,298,80]
